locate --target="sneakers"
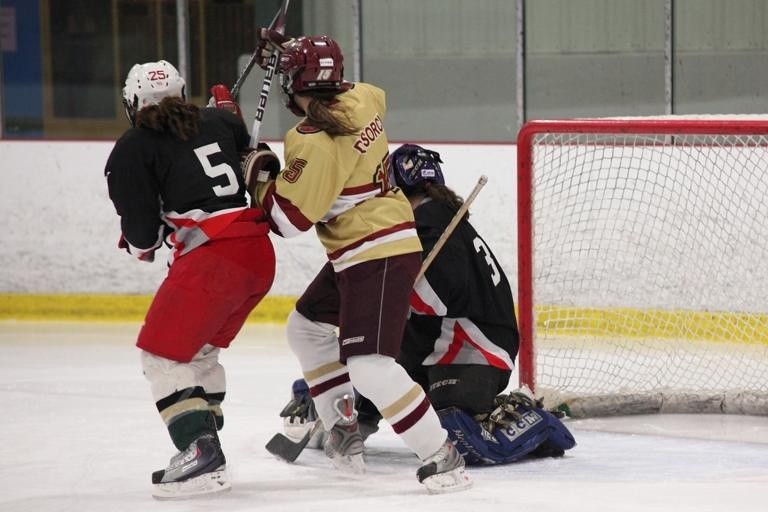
[323,396,365,458]
[416,436,466,483]
[152,431,227,484]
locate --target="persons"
[102,58,279,489]
[201,27,468,485]
[278,142,577,470]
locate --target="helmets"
[278,35,356,117]
[389,144,445,194]
[121,60,188,124]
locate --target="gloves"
[206,84,242,118]
[254,25,293,74]
[239,142,281,196]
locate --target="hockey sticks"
[265,176,490,463]
